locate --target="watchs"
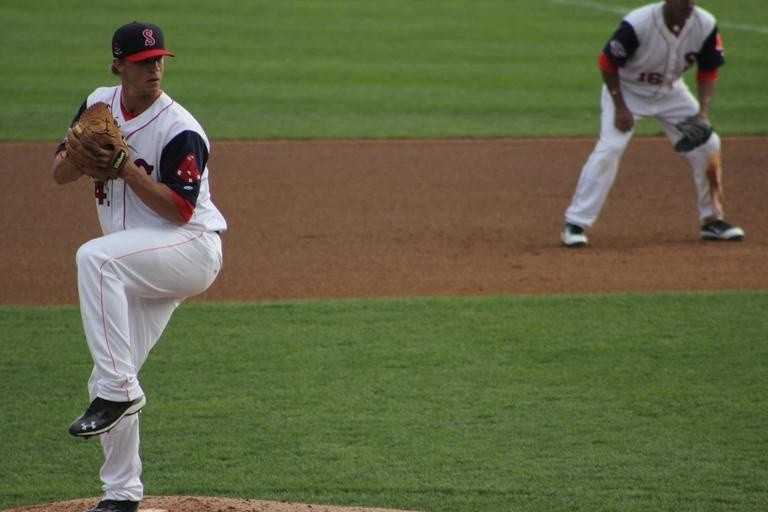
[610,87,621,97]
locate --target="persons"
[52,20,228,512]
[561,0,746,246]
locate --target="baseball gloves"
[673,114,713,152]
[65,101,130,182]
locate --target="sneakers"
[88,500,140,512]
[68,395,147,436]
[699,219,746,240]
[560,222,588,247]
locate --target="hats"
[112,20,175,63]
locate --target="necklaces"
[665,11,689,33]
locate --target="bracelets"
[699,100,711,107]
